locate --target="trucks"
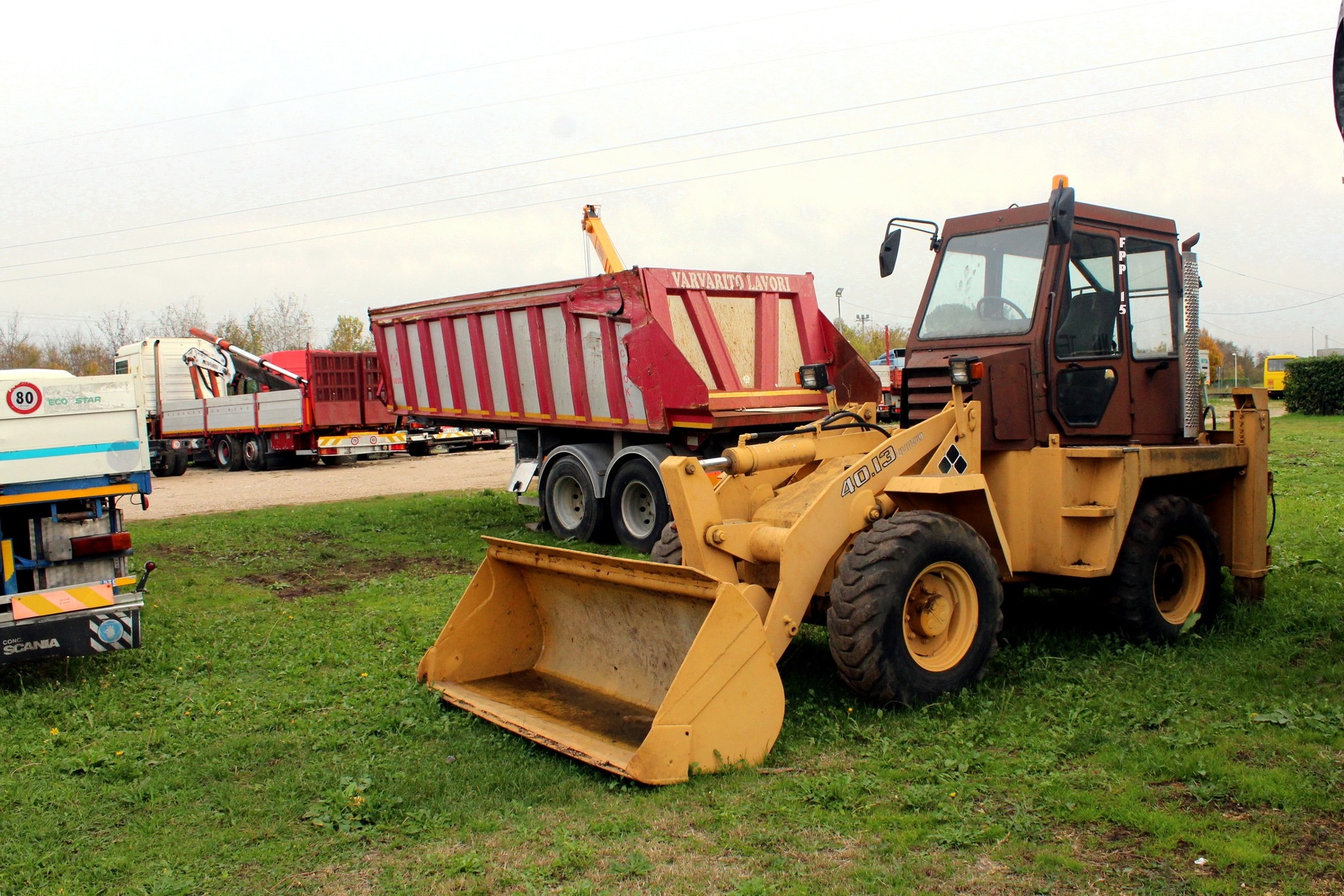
[1,367,159,671]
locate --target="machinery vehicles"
[414,169,1274,785]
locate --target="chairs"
[1056,291,1121,358]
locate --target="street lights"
[856,313,873,338]
[1231,353,1237,388]
[836,287,844,333]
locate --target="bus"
[1262,354,1300,399]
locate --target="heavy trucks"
[112,337,519,478]
